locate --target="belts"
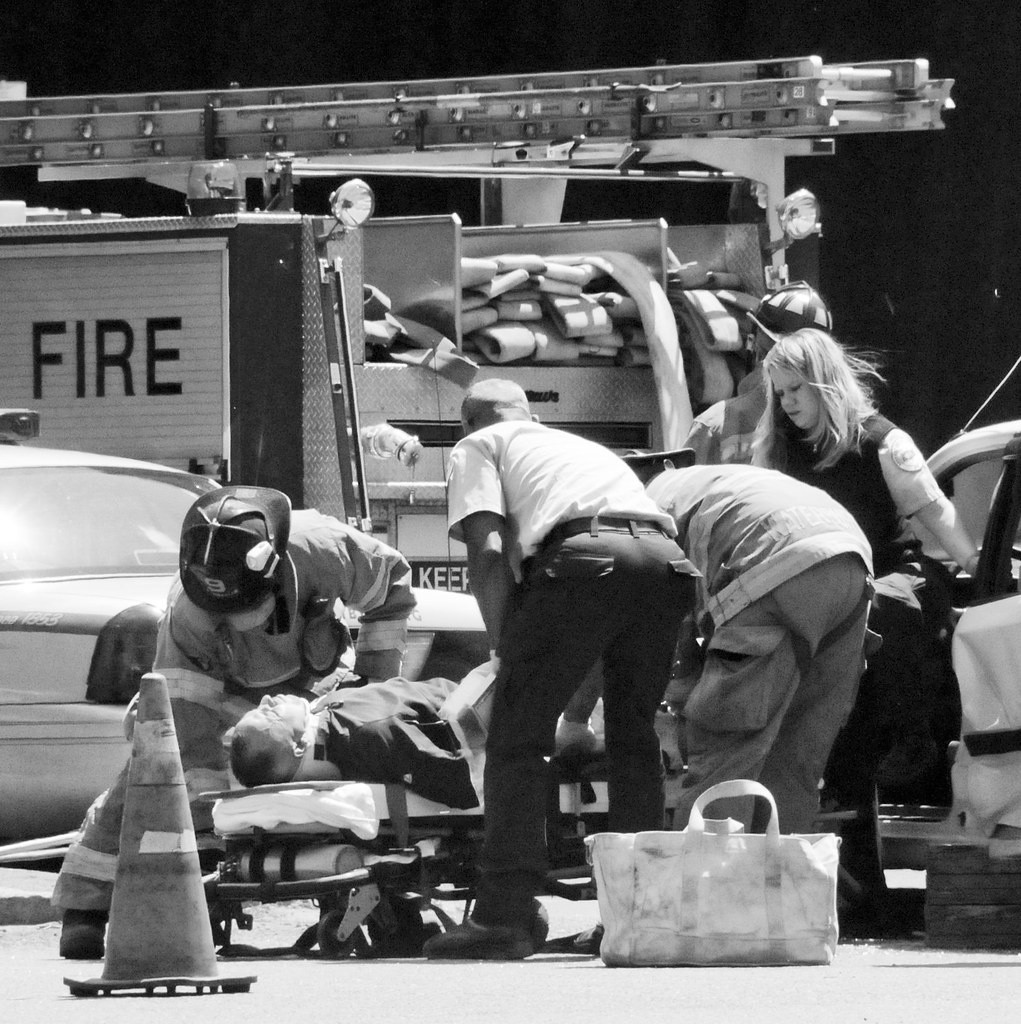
[538,516,664,548]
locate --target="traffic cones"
[60,660,260,998]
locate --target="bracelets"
[965,552,981,574]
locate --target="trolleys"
[201,777,695,961]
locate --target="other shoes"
[423,914,534,960]
[58,909,109,962]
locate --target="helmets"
[746,281,833,343]
[179,485,292,614]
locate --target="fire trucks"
[1,55,957,700]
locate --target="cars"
[886,418,1021,861]
[1,442,493,886]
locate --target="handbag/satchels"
[582,780,844,968]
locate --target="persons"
[623,441,878,845]
[426,378,705,979]
[49,482,419,965]
[689,278,1016,943]
